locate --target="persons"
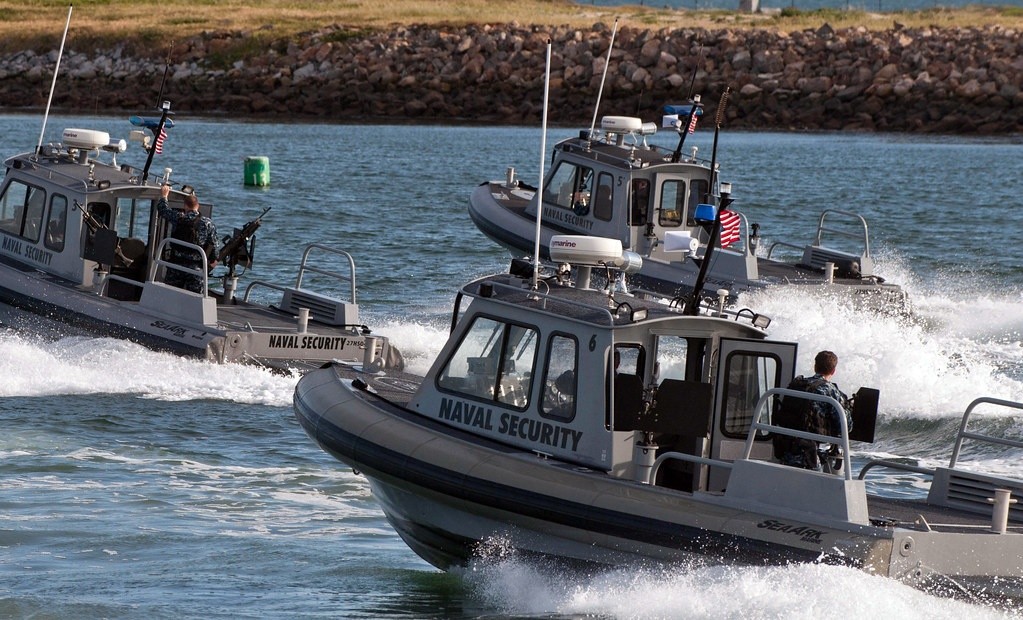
[157,186,220,294]
[633,178,647,210]
[596,185,611,217]
[773,350,853,474]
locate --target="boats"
[0,3,404,377]
[288,36,1023,612]
[466,18,914,330]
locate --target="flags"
[689,115,697,134]
[155,129,167,154]
[719,210,741,249]
